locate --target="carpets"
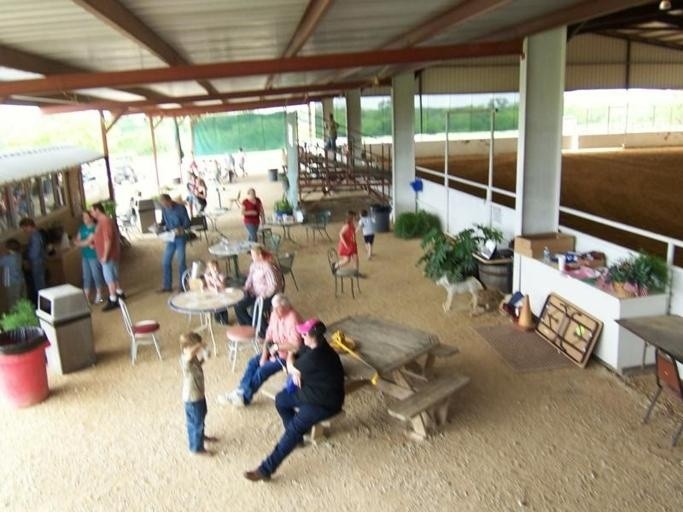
[472,320,580,375]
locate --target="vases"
[478,252,511,295]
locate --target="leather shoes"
[244,471,270,481]
[102,293,125,311]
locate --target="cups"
[558,255,566,270]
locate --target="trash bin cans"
[0,326,50,410]
[134,199,156,234]
[369,203,392,233]
[267,169,277,182]
[35,283,97,375]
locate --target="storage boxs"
[513,232,575,257]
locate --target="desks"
[611,313,682,368]
[208,239,263,286]
[301,315,443,433]
[167,287,244,360]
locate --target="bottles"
[542,246,550,263]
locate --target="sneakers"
[217,388,245,407]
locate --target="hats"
[296,319,326,336]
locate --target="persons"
[153,192,191,293]
[223,293,348,486]
[170,145,267,294]
[177,332,220,459]
[329,208,377,279]
[0,203,132,317]
[234,243,283,338]
[322,113,339,154]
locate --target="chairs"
[114,156,301,248]
[327,248,361,299]
[305,210,334,245]
[117,298,163,364]
[263,232,280,262]
[179,266,219,334]
[275,252,301,293]
[640,347,681,448]
[225,294,263,375]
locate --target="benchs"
[389,371,471,440]
[261,382,346,445]
[403,327,460,379]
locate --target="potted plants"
[603,256,634,298]
[0,298,50,410]
[625,249,672,297]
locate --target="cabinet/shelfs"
[510,251,671,370]
